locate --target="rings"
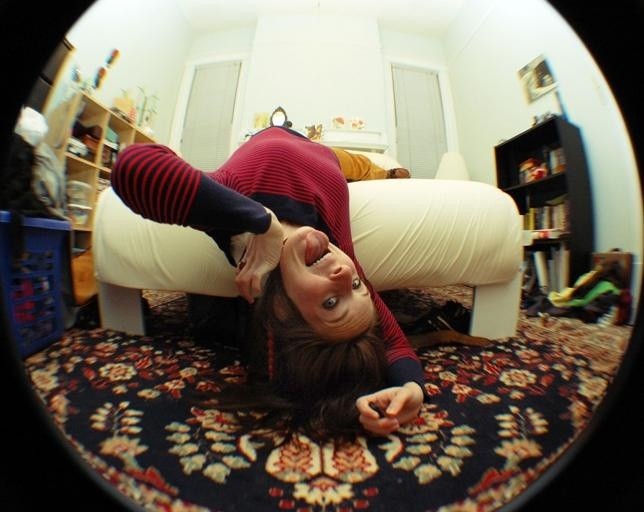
[236,259,247,270]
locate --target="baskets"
[1,209,71,359]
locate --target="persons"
[107,122,423,438]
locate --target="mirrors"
[269,106,292,129]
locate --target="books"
[521,148,570,294]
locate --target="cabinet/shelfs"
[494,119,596,289]
[46,92,159,250]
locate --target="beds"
[92,129,525,341]
